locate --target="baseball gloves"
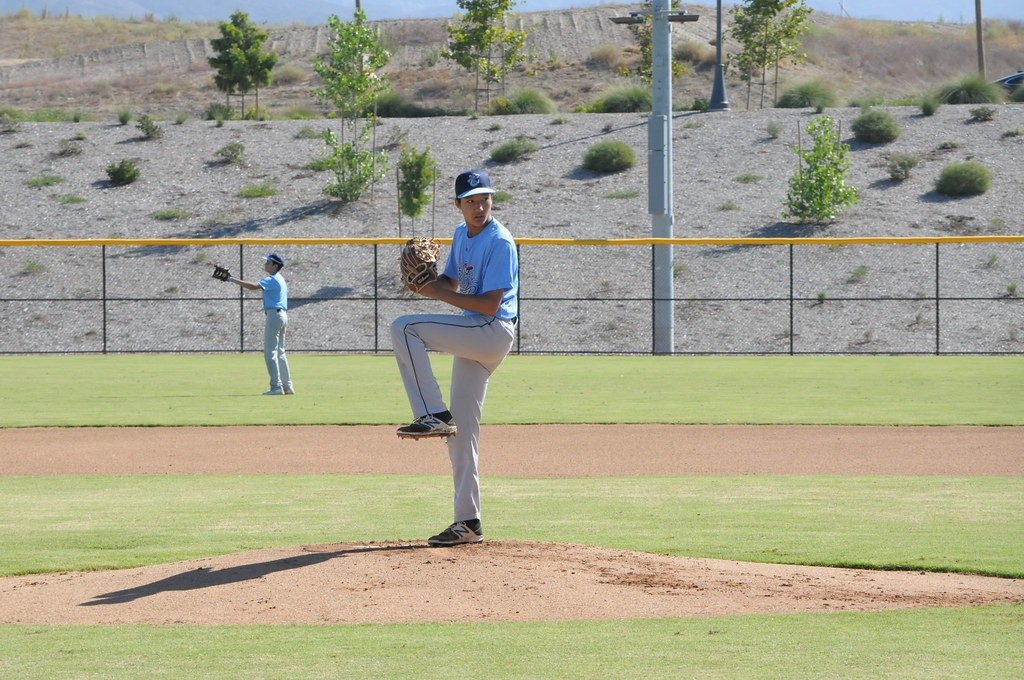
[211,264,232,283]
[400,236,444,294]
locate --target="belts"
[511,317,516,323]
[266,309,281,314]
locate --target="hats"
[455,172,495,198]
[263,253,284,267]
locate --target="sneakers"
[427,519,483,546]
[263,387,286,395]
[396,410,457,440]
[284,385,294,394]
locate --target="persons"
[212,253,295,395]
[390,170,519,545]
